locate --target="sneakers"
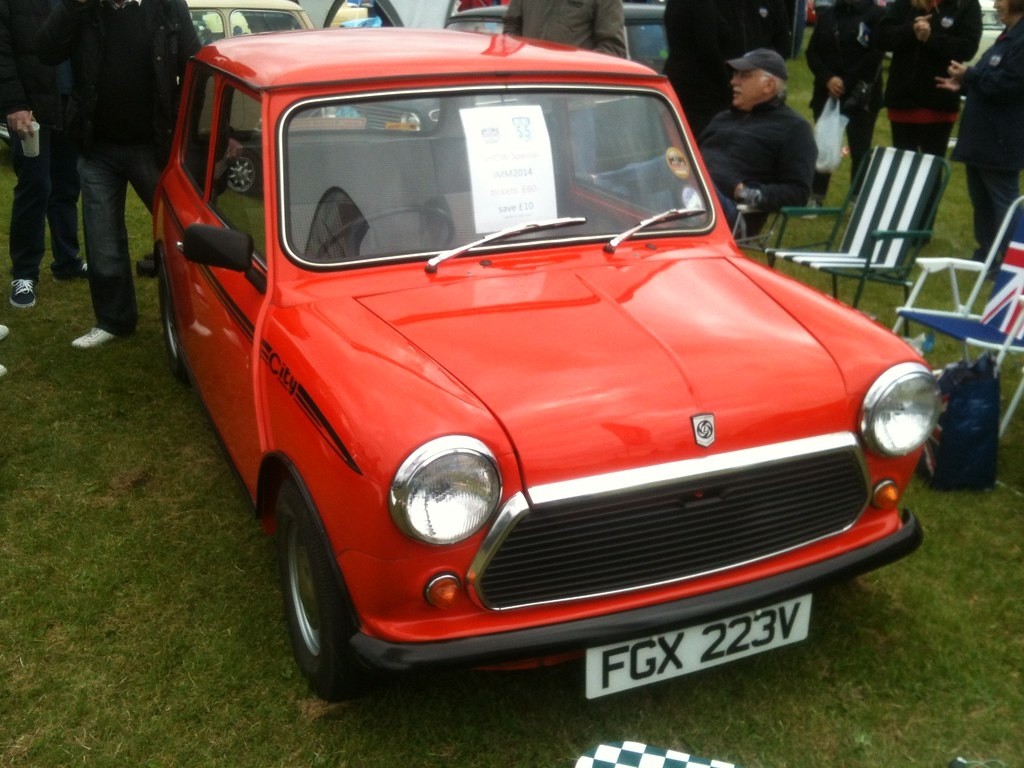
[72,327,116,349]
[9,278,37,308]
[52,262,89,283]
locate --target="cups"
[21,121,41,157]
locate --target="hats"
[726,48,789,80]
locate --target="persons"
[662,0,792,142]
[504,0,625,175]
[807,0,1024,276]
[0,0,202,376]
[593,49,817,242]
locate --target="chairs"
[761,145,952,338]
[727,199,784,270]
[218,132,481,261]
[892,194,1024,437]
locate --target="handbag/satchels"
[916,352,999,488]
[841,76,884,115]
[813,94,849,172]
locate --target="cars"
[178,1,670,198]
[152,25,946,709]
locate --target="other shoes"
[849,202,855,216]
[0,324,10,339]
[0,365,7,376]
[972,254,986,263]
[913,238,931,244]
[801,198,822,218]
